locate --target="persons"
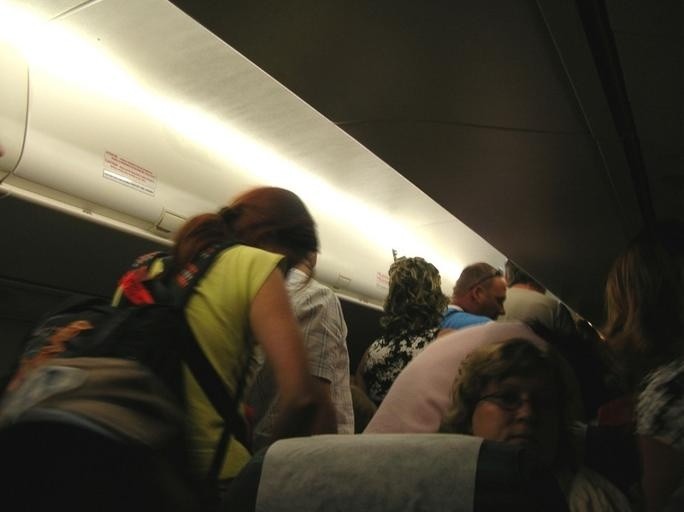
[348,252,615,434]
[240,243,355,448]
[602,216,683,510]
[119,183,321,486]
[444,339,633,511]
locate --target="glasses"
[477,388,561,413]
[470,269,503,290]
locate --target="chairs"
[220,434,525,510]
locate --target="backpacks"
[1,242,246,509]
